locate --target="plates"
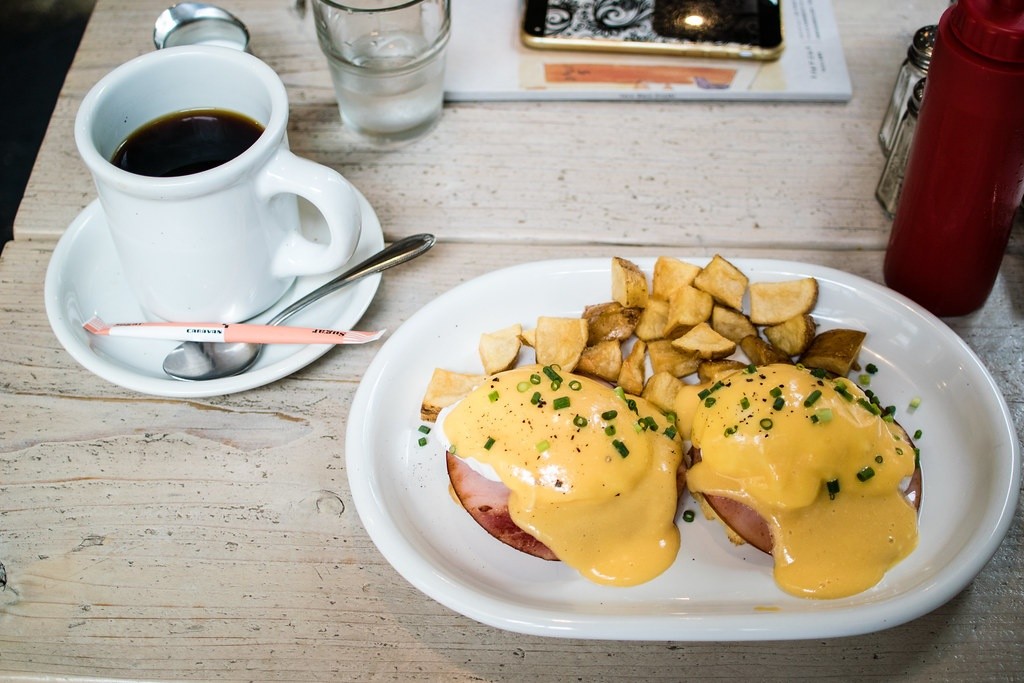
[345,255,1021,644]
[43,153,386,401]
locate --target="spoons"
[163,232,437,385]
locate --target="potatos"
[418,256,868,416]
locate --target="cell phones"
[521,0,785,61]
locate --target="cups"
[309,0,453,153]
[73,43,361,327]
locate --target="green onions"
[417,363,922,522]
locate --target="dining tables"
[0,0,1024,683]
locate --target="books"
[421,0,853,102]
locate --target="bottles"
[883,0,1024,319]
[879,23,937,158]
[874,77,928,219]
